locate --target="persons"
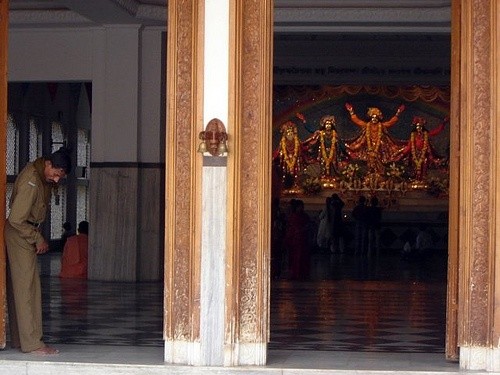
[3,150,73,353]
[273,121,309,178]
[282,199,311,280]
[61,222,73,246]
[352,195,382,258]
[400,224,433,264]
[60,221,88,280]
[345,103,406,161]
[297,111,351,181]
[405,116,450,180]
[316,194,345,254]
